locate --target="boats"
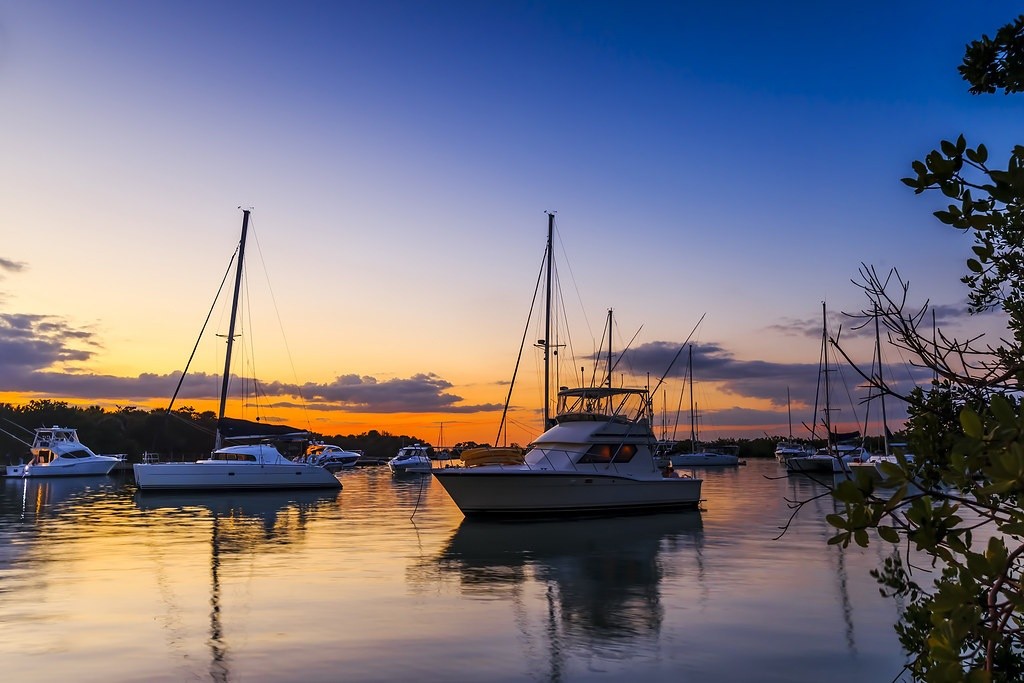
[289,439,360,470]
[388,443,433,476]
[5,423,128,477]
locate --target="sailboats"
[659,344,746,466]
[132,207,345,494]
[775,296,920,485]
[435,211,706,521]
